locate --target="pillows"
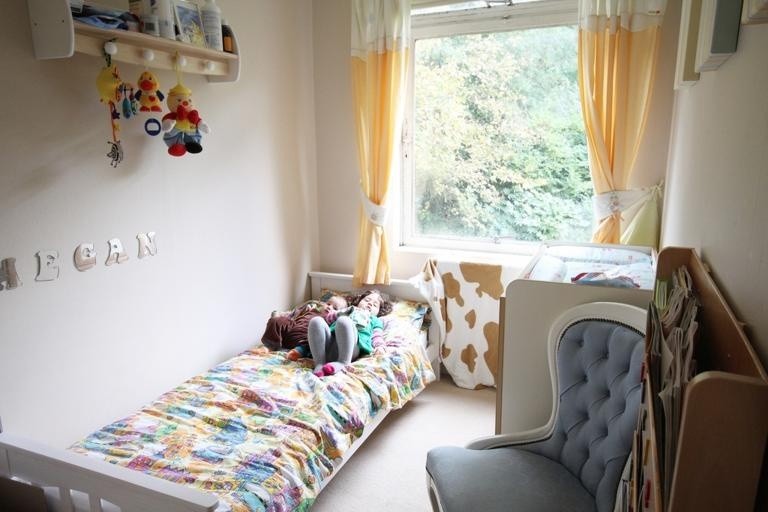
[319,287,431,347]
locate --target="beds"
[0,271,441,512]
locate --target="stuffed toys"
[160,83,210,157]
[97,64,137,169]
[133,71,164,113]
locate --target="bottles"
[200,0,233,53]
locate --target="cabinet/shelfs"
[622,244,768,512]
[495,238,657,434]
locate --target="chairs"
[426,301,649,512]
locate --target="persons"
[305,288,393,378]
[250,296,348,361]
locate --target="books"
[611,265,702,512]
[171,1,209,50]
